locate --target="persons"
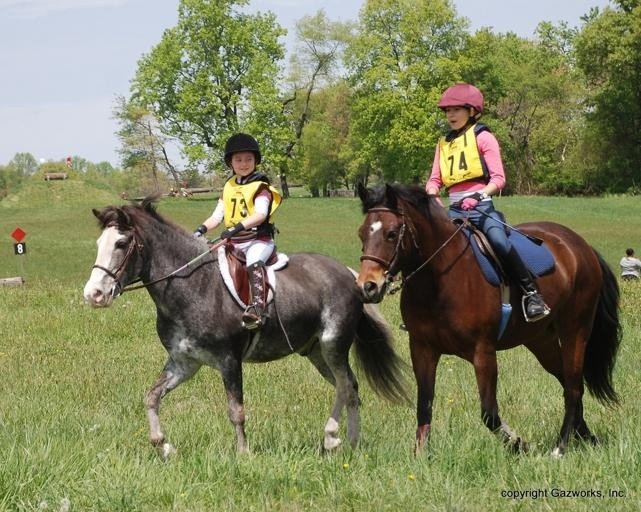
[193,130,285,335]
[616,250,641,282]
[422,80,546,332]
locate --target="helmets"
[437,82,484,113]
[224,133,261,167]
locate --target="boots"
[243,261,268,329]
[502,244,545,318]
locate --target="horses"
[352,179,625,460]
[83,193,415,465]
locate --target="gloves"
[221,223,244,243]
[459,192,488,210]
